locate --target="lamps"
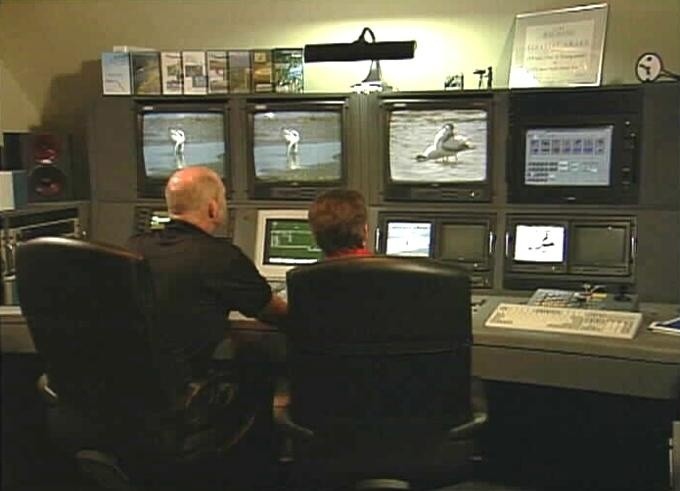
[303,27,418,93]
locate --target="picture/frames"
[508,3,610,88]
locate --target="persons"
[126,167,292,490]
[273,189,474,432]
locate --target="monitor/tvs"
[380,218,433,259]
[147,208,171,228]
[242,101,351,201]
[506,220,566,263]
[521,122,614,188]
[377,95,493,204]
[439,220,486,262]
[573,224,626,268]
[133,102,233,198]
[254,210,324,281]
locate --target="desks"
[0,283,680,399]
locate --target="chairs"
[14,236,273,491]
[279,256,488,489]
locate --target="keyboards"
[484,302,643,340]
[528,287,639,313]
